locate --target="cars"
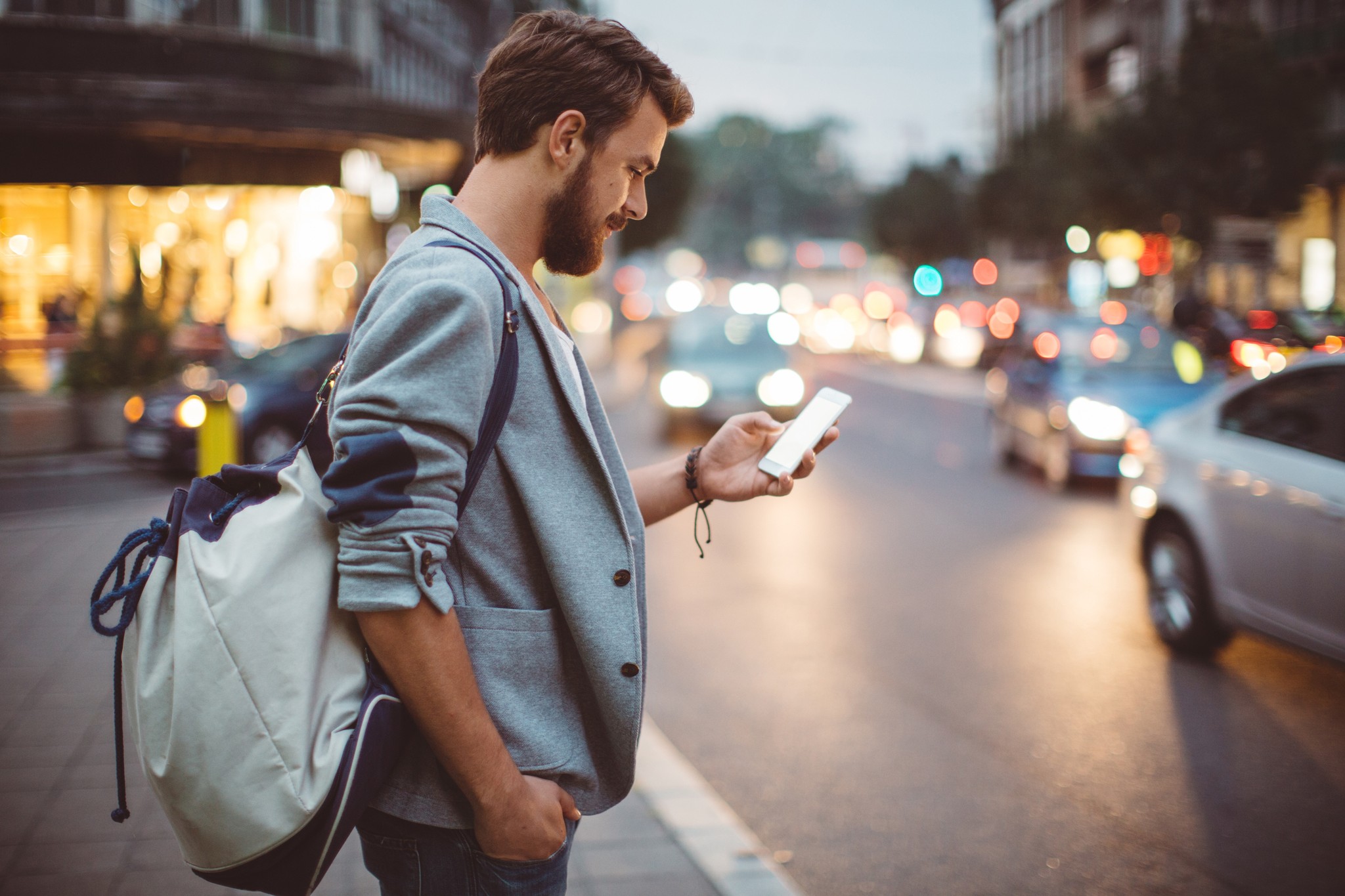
[125,330,346,479]
[659,303,804,437]
[986,312,1227,497]
[1116,350,1343,670]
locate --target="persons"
[42,295,79,334]
[319,8,842,896]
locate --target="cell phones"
[757,386,853,479]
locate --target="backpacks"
[90,238,521,896]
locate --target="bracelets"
[685,444,717,559]
